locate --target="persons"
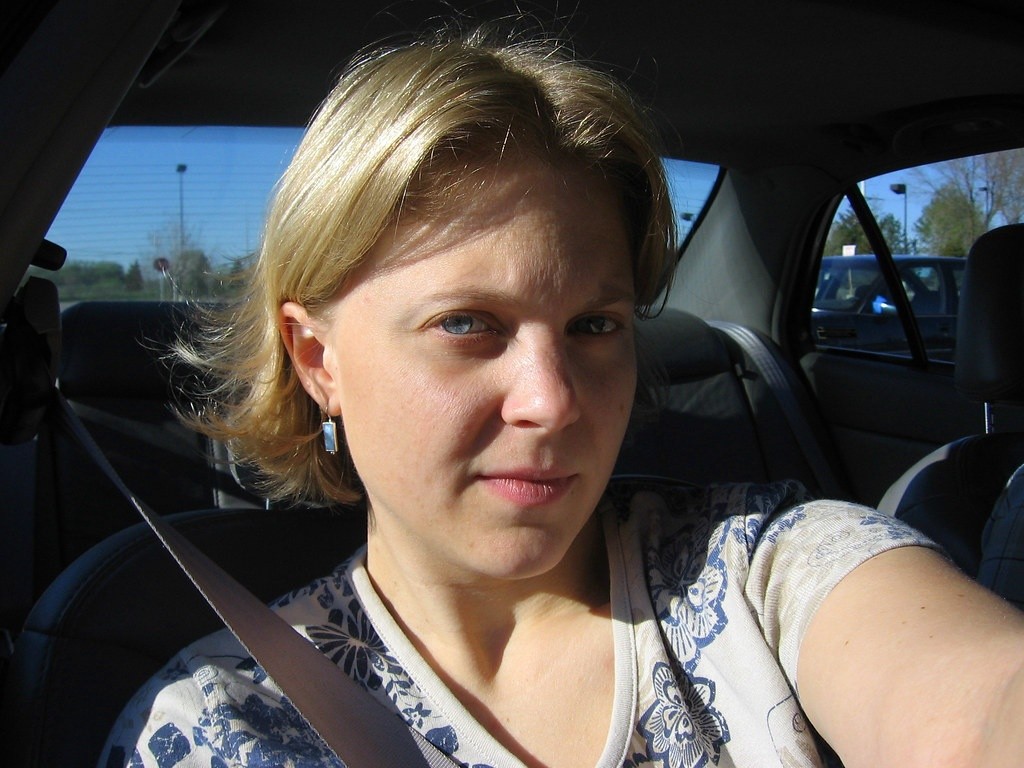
[100,39,1024,767]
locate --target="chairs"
[20,363,366,768]
[877,221,1024,615]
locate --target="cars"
[809,252,967,362]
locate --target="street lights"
[890,184,908,254]
[176,162,189,302]
[978,185,990,224]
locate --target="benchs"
[36,301,852,578]
